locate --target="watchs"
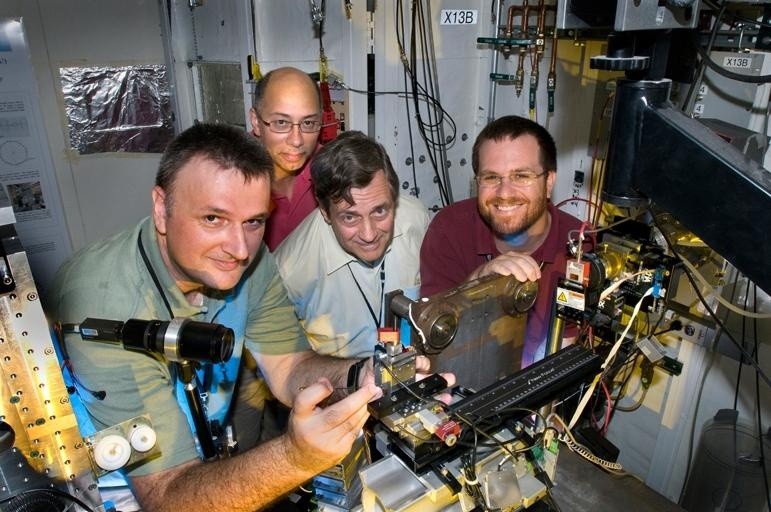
[347,357,370,394]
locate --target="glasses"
[252,108,322,134]
[473,170,548,187]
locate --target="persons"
[272,131,436,358]
[229,66,323,454]
[420,115,598,370]
[44,123,456,512]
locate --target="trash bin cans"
[677,407,771,512]
